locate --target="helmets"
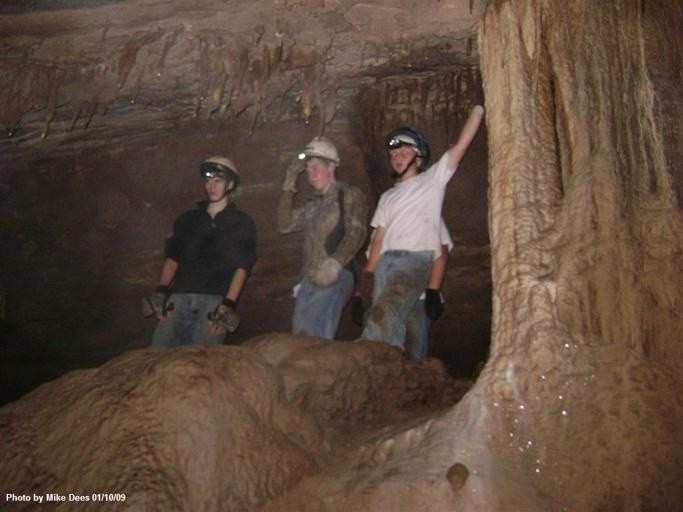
[305,135,340,163]
[200,156,239,192]
[386,127,430,170]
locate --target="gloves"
[207,298,241,334]
[282,156,306,192]
[140,285,172,321]
[309,257,343,287]
[424,288,443,320]
[351,296,364,327]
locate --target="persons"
[352,216,454,361]
[147,154,258,347]
[365,80,486,352]
[268,135,370,341]
